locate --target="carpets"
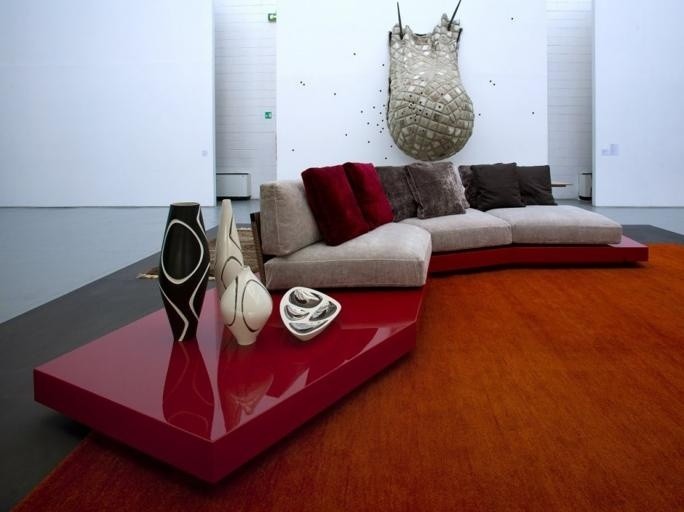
[624,224,684,244]
[135,227,259,281]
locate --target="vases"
[219,265,273,345]
[158,201,210,341]
[215,199,243,304]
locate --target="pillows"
[301,164,369,246]
[459,163,503,204]
[375,166,418,222]
[471,162,525,212]
[517,165,557,205]
[342,162,394,225]
[404,161,466,219]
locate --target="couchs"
[249,161,622,290]
